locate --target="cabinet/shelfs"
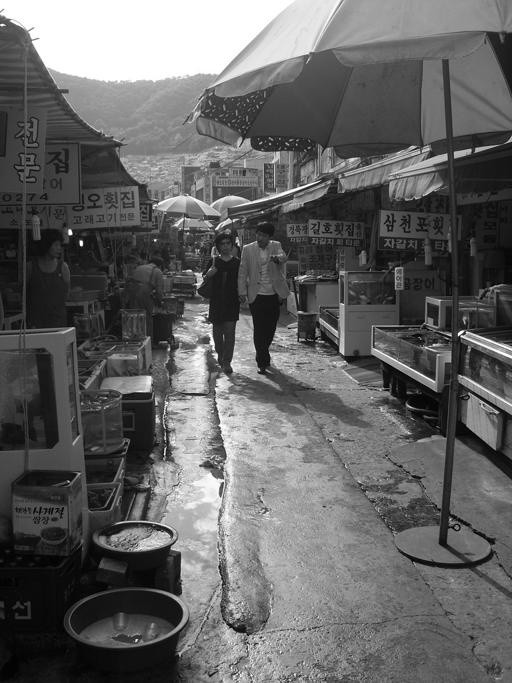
[458,388,512,460]
[341,270,400,357]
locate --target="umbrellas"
[214,217,245,231]
[208,193,253,219]
[151,192,221,257]
[183,0,511,544]
[168,214,215,231]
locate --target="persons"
[197,233,239,372]
[29,229,71,327]
[124,256,165,309]
[235,220,291,374]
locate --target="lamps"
[131,230,138,248]
[470,235,480,258]
[31,207,42,241]
[446,226,452,254]
[423,196,434,266]
[62,221,71,246]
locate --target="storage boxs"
[99,374,156,447]
[82,457,130,533]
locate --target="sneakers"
[217,350,233,374]
[255,349,271,374]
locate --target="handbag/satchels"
[196,274,213,300]
[131,279,157,303]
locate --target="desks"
[1,307,28,330]
[64,299,96,314]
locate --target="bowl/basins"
[91,520,178,568]
[64,587,189,670]
[39,525,66,547]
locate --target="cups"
[113,613,129,630]
[143,622,160,641]
